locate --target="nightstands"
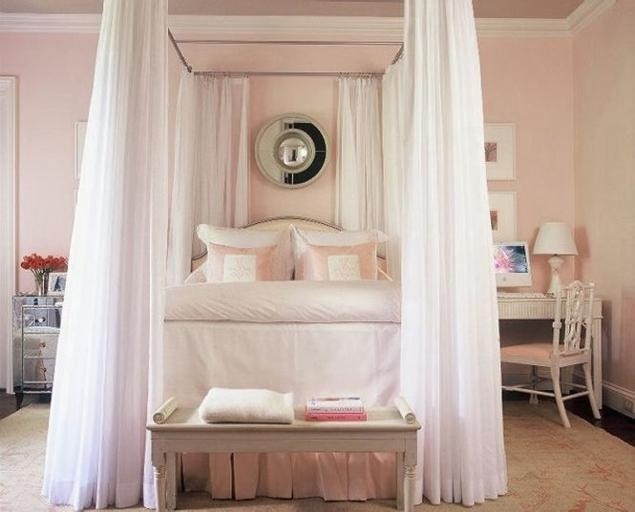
[8,292,65,408]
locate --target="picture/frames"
[483,122,518,245]
[71,118,89,180]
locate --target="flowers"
[18,252,67,283]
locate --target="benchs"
[144,393,424,507]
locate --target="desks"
[494,290,606,412]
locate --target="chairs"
[499,279,606,430]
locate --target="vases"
[32,272,51,297]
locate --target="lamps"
[530,220,580,296]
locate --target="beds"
[159,214,409,507]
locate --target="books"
[305,410,368,421]
[305,394,367,414]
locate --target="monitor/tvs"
[493,241,533,287]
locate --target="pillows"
[193,222,383,283]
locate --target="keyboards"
[497,292,546,299]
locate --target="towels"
[198,386,297,426]
